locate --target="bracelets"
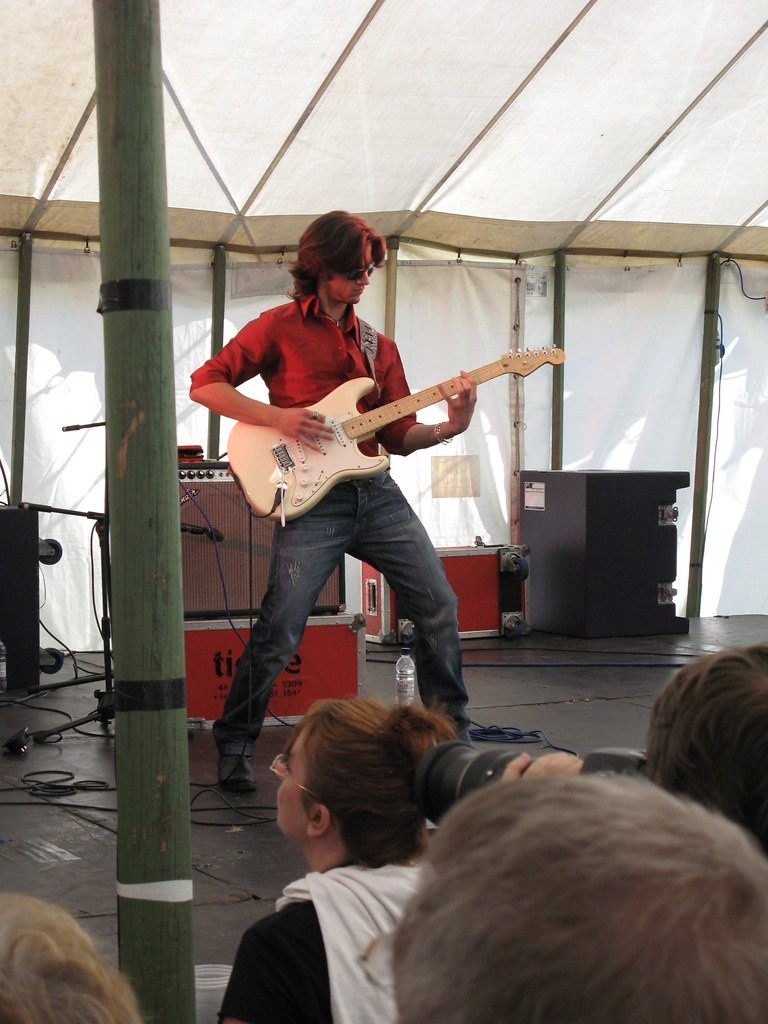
[434,422,454,445]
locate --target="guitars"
[226,341,565,525]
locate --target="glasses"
[342,261,376,280]
[269,754,336,830]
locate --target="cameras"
[413,742,648,825]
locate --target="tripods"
[19,504,210,745]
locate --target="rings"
[312,411,318,420]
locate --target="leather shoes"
[217,755,257,791]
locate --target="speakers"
[0,504,40,691]
[178,462,347,618]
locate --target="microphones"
[204,527,223,541]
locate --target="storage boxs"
[185,614,366,736]
[361,545,526,645]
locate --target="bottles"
[395,649,415,707]
[0,640,8,694]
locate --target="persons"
[216,644,768,1024]
[189,212,477,790]
[0,890,141,1024]
